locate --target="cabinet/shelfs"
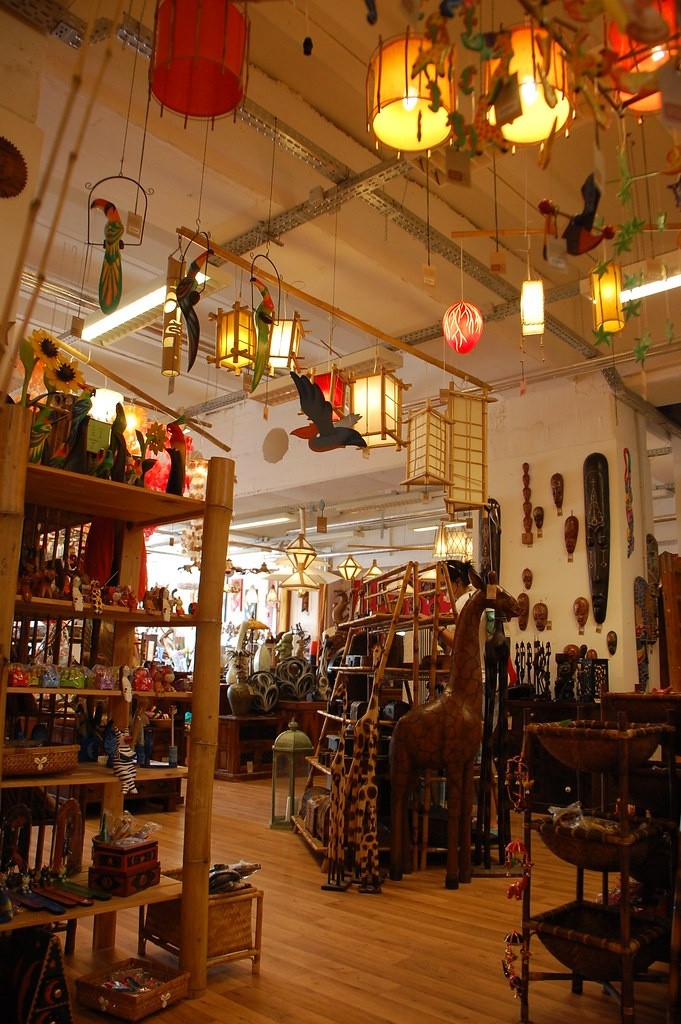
[0,404,681,1024]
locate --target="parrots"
[32,383,187,498]
[90,198,125,315]
[250,277,274,394]
[176,249,214,374]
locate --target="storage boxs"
[90,837,160,865]
[88,863,161,897]
[138,867,265,976]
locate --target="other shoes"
[480,833,498,845]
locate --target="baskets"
[528,719,669,773]
[531,900,663,982]
[3,740,80,775]
[76,958,190,1022]
[537,805,651,871]
[145,866,256,953]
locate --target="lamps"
[444,379,498,529]
[367,34,457,159]
[265,310,311,377]
[297,363,357,425]
[147,0,249,119]
[610,0,681,113]
[346,366,413,458]
[520,237,546,335]
[91,374,146,453]
[161,256,186,378]
[206,302,256,376]
[283,535,382,581]
[589,240,626,337]
[441,243,482,355]
[79,258,228,348]
[484,26,574,152]
[432,518,475,563]
[400,402,455,503]
[579,248,681,304]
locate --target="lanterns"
[442,381,498,518]
[207,300,257,376]
[589,263,626,335]
[146,1,251,131]
[482,17,579,154]
[266,310,311,376]
[603,0,681,124]
[343,365,412,459]
[297,363,357,423]
[364,25,457,159]
[399,398,456,492]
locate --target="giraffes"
[387,565,523,890]
[320,644,384,895]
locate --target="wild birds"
[290,371,368,451]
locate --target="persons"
[436,560,498,835]
